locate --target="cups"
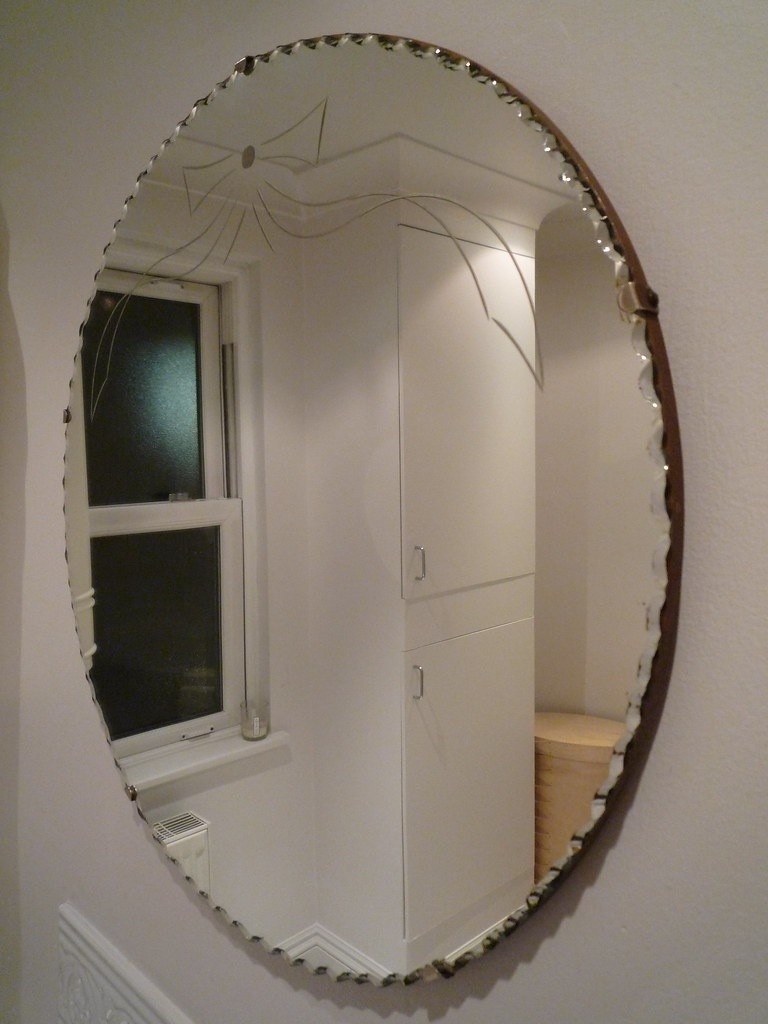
[240,698,270,741]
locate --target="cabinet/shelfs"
[393,228,576,938]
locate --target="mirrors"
[60,30,683,989]
